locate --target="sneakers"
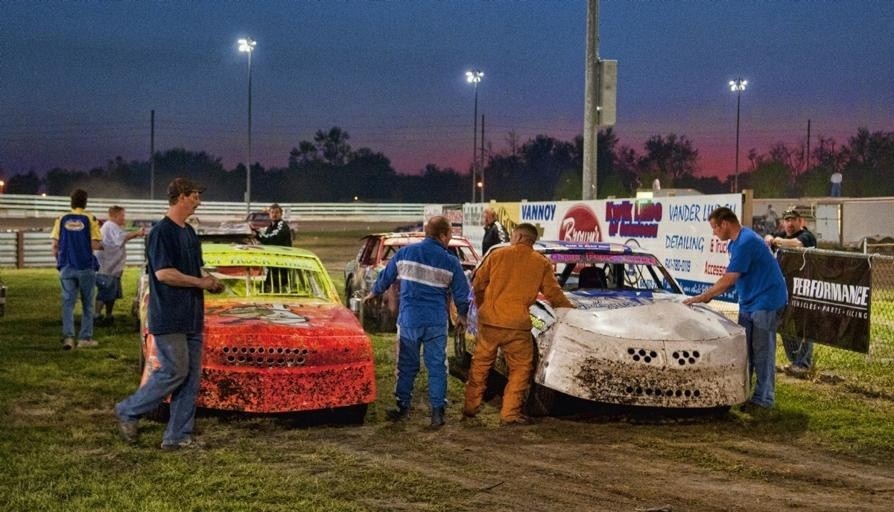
[741,402,764,412]
[431,414,444,428]
[460,413,488,427]
[62,337,74,350]
[385,404,410,422]
[160,436,208,452]
[77,339,99,348]
[93,315,115,328]
[500,413,536,429]
[114,403,139,443]
[777,362,812,376]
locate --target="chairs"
[578,267,608,290]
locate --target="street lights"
[465,67,486,203]
[236,36,257,223]
[728,77,748,193]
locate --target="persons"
[463,223,576,425]
[764,209,817,373]
[50,188,104,351]
[363,215,471,428]
[683,207,789,414]
[93,206,145,328]
[113,179,226,451]
[249,204,292,294]
[483,208,510,256]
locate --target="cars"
[136,239,376,417]
[343,231,481,329]
[452,239,754,408]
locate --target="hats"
[783,209,800,219]
[166,177,208,198]
